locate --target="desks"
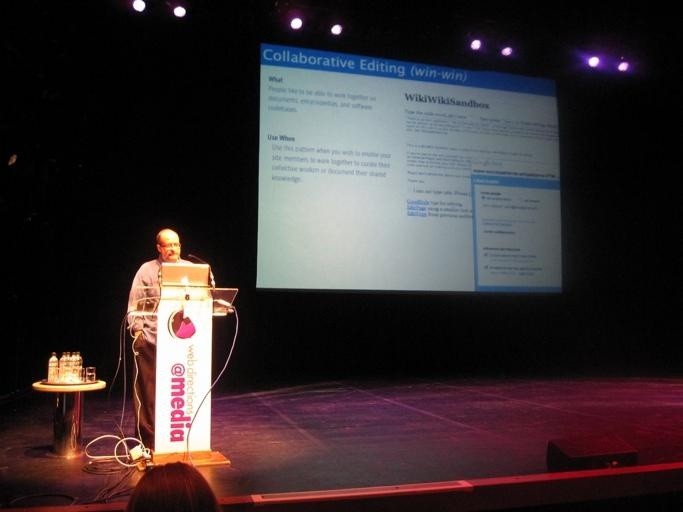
[33,378,106,458]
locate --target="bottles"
[48,351,83,383]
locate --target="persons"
[126,460,221,511]
[124,228,193,450]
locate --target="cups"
[83,367,96,382]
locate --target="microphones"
[188,252,216,288]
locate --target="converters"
[129,445,142,461]
[218,298,231,307]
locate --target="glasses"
[160,242,182,248]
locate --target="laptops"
[161,263,210,286]
[207,288,239,310]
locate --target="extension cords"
[143,452,151,459]
